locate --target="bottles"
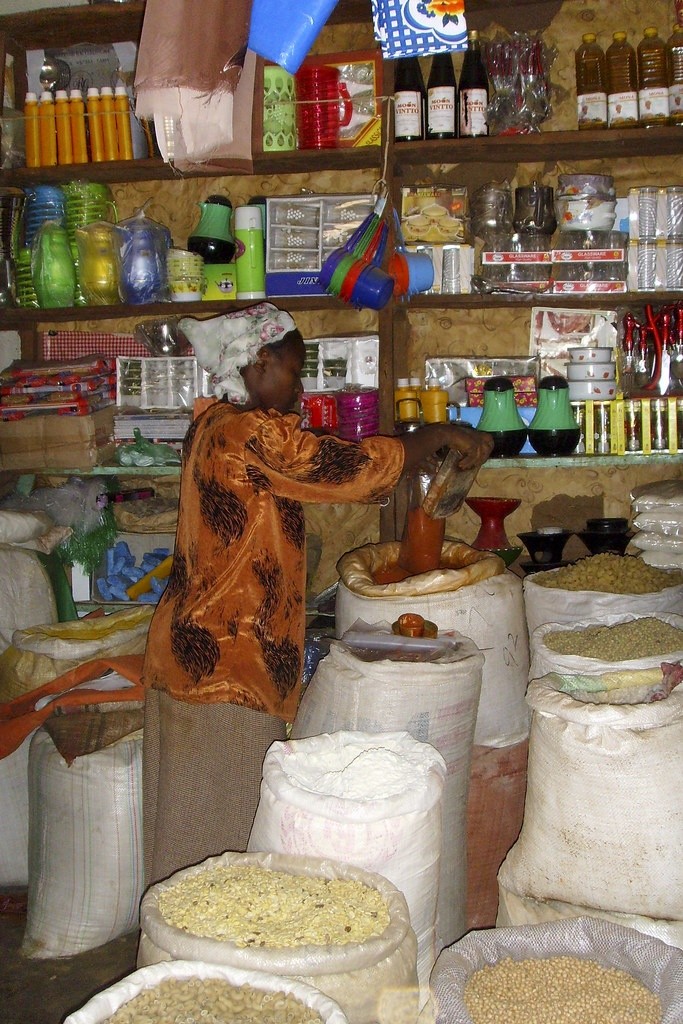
[458,30,493,138]
[575,34,609,130]
[25,87,133,171]
[667,23,683,127]
[604,31,640,129]
[234,203,266,300]
[394,53,426,141]
[425,51,459,139]
[394,377,449,429]
[637,27,671,129]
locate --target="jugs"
[295,66,353,150]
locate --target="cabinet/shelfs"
[0,0,683,544]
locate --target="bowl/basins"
[464,493,633,573]
[552,173,617,232]
[563,347,618,402]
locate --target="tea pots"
[470,178,514,235]
[473,375,581,458]
[513,180,557,234]
[16,186,240,305]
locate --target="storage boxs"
[64,558,91,603]
[400,183,475,246]
[264,195,378,298]
[0,404,115,471]
[91,530,176,605]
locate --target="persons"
[143,302,495,887]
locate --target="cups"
[417,248,460,294]
[335,386,379,442]
[637,187,683,291]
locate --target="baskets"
[113,478,181,533]
